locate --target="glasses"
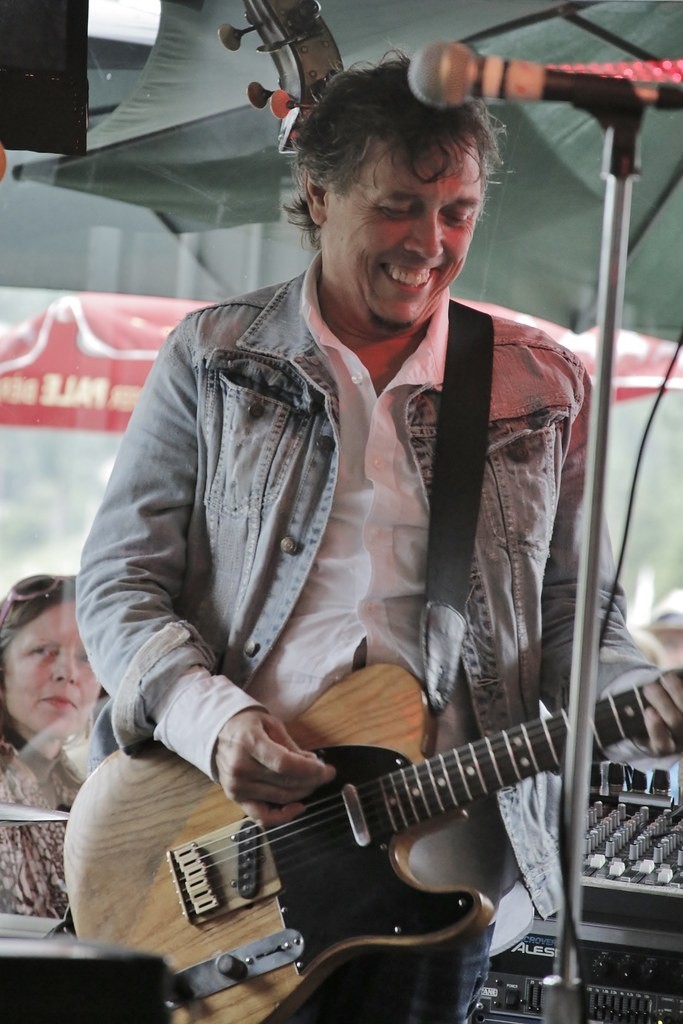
[1,576,64,627]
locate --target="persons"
[75,60,683,1024]
[0,575,109,936]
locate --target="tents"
[0,291,683,433]
[13,0,683,343]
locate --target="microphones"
[408,40,683,111]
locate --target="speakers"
[0,0,90,153]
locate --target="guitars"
[63,664,683,1023]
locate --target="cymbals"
[0,803,69,824]
